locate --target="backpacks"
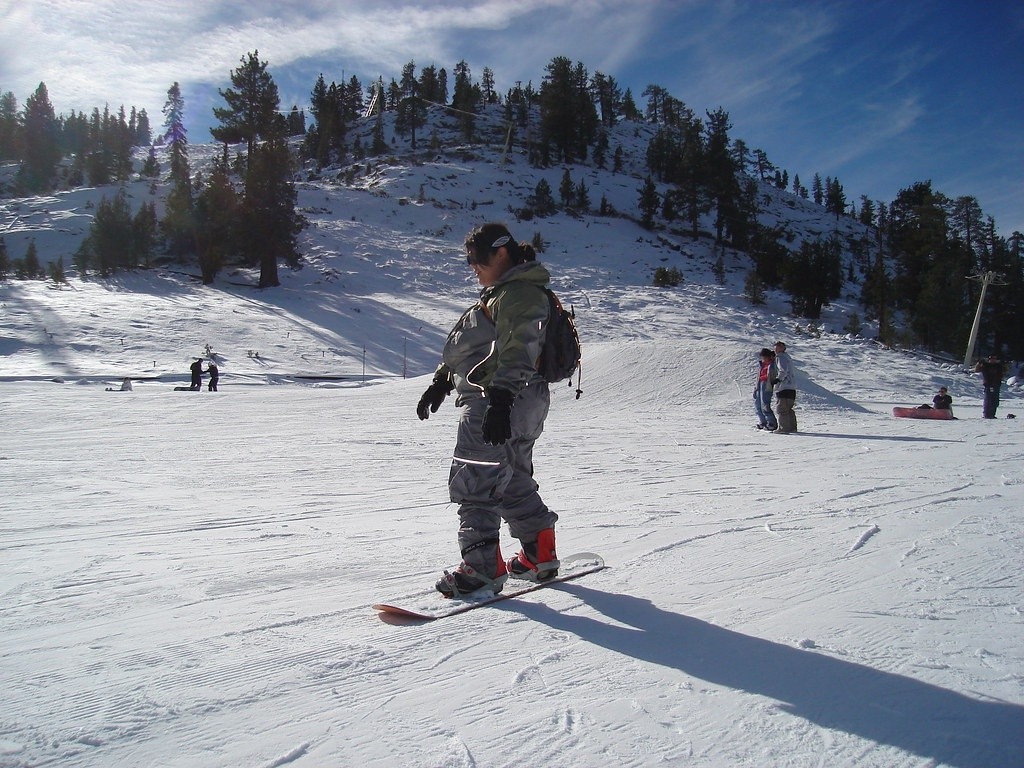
[537,284,581,399]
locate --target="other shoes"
[772,427,789,434]
[755,423,764,429]
[509,542,539,575]
[436,564,486,595]
[763,423,777,430]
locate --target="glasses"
[939,391,944,393]
[467,252,483,265]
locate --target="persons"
[917,386,953,418]
[121,377,132,390]
[189,358,219,392]
[752,340,799,435]
[417,220,559,598]
[974,352,1010,420]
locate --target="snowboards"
[372,549,606,622]
[174,386,201,391]
[890,406,953,421]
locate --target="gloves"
[417,379,446,420]
[481,387,514,446]
